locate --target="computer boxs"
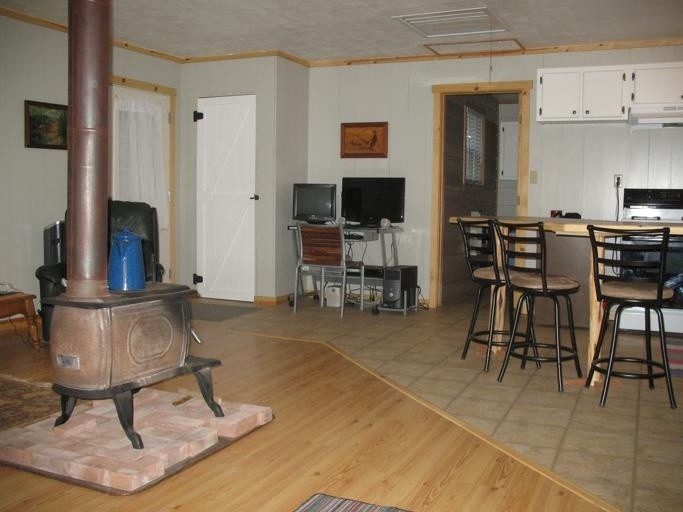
[384,265,417,311]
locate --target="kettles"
[107,225,146,292]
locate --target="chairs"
[34,200,164,340]
[490,220,583,393]
[293,222,365,320]
[456,217,542,370]
[585,224,677,410]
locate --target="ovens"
[623,188,683,309]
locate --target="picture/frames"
[24,100,68,150]
[341,122,388,159]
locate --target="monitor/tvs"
[292,183,336,224]
[341,177,406,227]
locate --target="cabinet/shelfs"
[498,121,518,180]
[535,61,629,123]
[629,61,683,124]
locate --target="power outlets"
[614,175,623,188]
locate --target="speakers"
[326,286,341,307]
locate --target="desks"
[0,292,41,350]
[288,225,403,313]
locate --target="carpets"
[292,493,414,512]
[0,373,91,432]
[665,338,683,378]
